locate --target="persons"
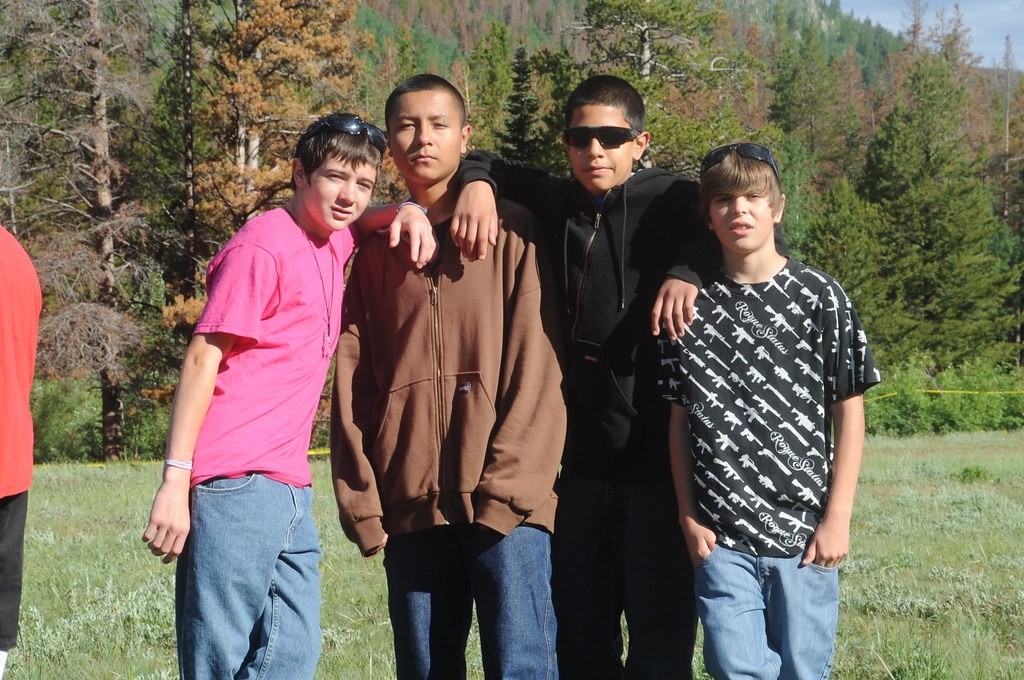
[333,72,570,680]
[635,139,883,680]
[0,225,46,677]
[144,109,442,680]
[448,70,723,680]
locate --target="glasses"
[562,125,640,150]
[700,144,780,183]
[297,113,387,154]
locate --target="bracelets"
[162,457,194,472]
